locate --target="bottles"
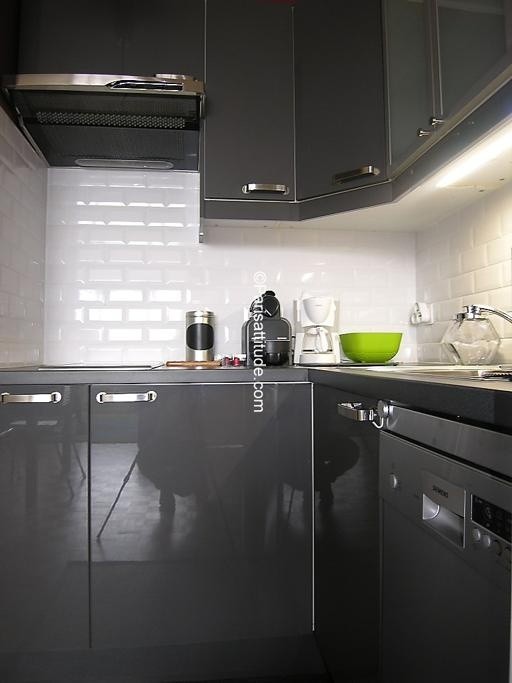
[440,315,501,365]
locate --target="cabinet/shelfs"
[203,2,298,223]
[293,2,396,222]
[2,382,316,653]
[384,2,512,213]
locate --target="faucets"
[464,304,512,327]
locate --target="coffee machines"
[293,295,341,366]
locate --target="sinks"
[365,366,504,380]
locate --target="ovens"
[374,399,512,682]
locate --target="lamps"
[433,121,511,188]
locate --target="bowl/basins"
[340,331,404,363]
